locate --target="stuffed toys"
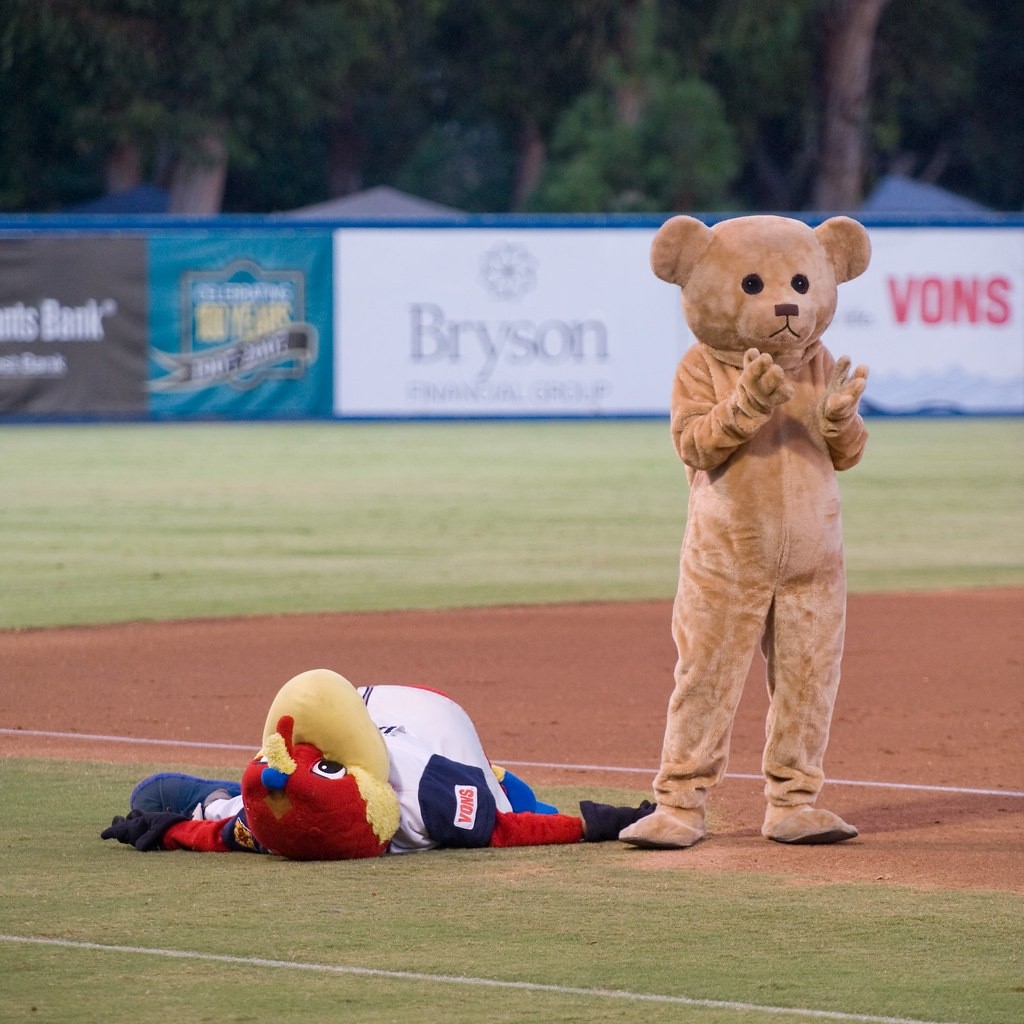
[100,667,659,862]
[621,215,872,848]
